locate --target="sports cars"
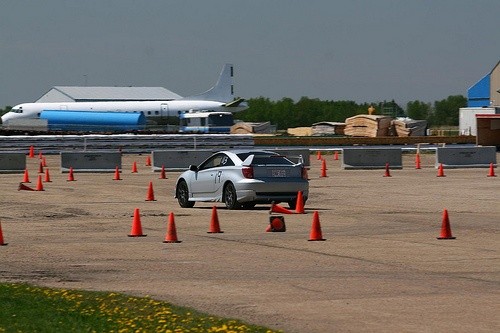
[174,148,310,210]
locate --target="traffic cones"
[36,175,45,191]
[436,162,446,177]
[292,191,308,215]
[414,152,422,170]
[382,163,393,177]
[158,163,169,180]
[486,163,498,177]
[17,182,36,191]
[145,155,153,167]
[131,161,139,173]
[436,208,458,240]
[206,205,225,234]
[127,207,147,237]
[24,169,32,183]
[162,212,182,243]
[65,166,78,182]
[316,150,338,178]
[268,201,293,215]
[28,145,53,183]
[307,211,327,242]
[145,179,158,201]
[0,217,9,246]
[112,166,124,180]
[265,215,286,233]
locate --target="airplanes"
[0,62,251,134]
